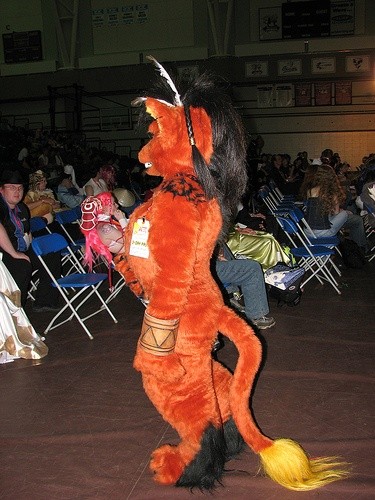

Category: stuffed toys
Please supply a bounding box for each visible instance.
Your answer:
[121,55,352,492]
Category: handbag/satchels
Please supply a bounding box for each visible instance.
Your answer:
[263,261,305,290]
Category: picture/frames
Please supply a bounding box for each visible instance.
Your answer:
[311,57,337,74]
[245,60,269,79]
[277,59,303,76]
[344,55,371,74]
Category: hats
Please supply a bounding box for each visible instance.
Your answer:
[59,172,73,182]
[0,169,27,185]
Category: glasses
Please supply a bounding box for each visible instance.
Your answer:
[4,185,24,192]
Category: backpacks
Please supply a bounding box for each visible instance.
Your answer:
[265,275,303,307]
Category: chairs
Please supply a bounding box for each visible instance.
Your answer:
[19,204,146,339]
[259,180,342,296]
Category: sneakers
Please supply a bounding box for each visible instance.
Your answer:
[248,316,276,330]
[230,297,245,313]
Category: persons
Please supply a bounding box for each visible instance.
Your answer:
[0,117,375,365]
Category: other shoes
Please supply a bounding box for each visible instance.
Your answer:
[31,301,62,313]
